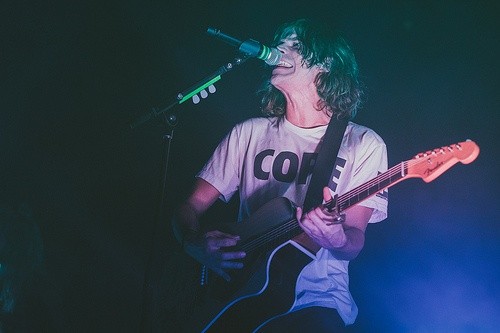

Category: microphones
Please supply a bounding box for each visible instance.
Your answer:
[206,26,281,65]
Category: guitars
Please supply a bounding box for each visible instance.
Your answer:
[181,137,481,331]
